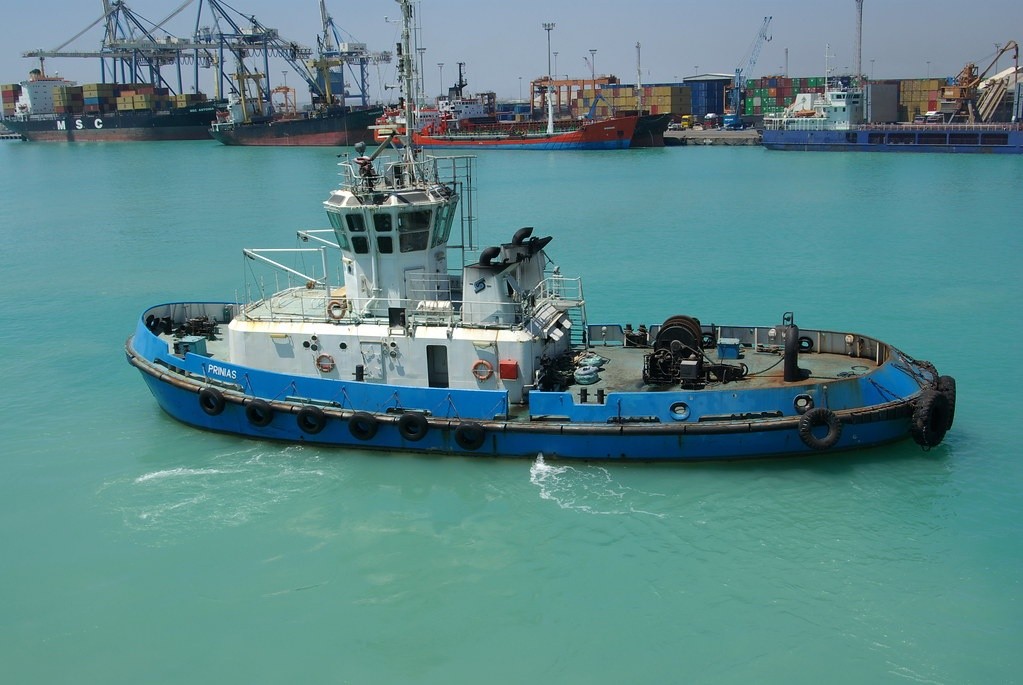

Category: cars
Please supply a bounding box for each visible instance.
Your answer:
[692,122,704,131]
[668,123,684,131]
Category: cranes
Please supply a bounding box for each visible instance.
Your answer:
[20,0,392,116]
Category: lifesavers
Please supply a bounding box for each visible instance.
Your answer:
[347,411,378,441]
[450,137,454,141]
[496,137,500,141]
[797,406,843,451]
[520,136,525,140]
[199,388,225,417]
[546,134,550,139]
[398,412,429,442]
[315,353,335,374]
[245,399,273,428]
[454,420,486,452]
[471,360,493,381]
[912,389,950,450]
[581,127,585,132]
[937,374,957,430]
[296,405,327,434]
[471,137,474,141]
[326,301,345,320]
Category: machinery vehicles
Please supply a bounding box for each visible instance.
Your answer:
[722,14,773,131]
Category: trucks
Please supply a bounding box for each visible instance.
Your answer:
[704,113,723,130]
[680,114,704,130]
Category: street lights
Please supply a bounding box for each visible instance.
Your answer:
[634,40,641,115]
[553,51,558,78]
[437,63,444,95]
[590,49,597,99]
[519,77,522,102]
[416,46,427,96]
[543,23,556,79]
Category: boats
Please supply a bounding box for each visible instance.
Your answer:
[366,0,677,151]
[207,1,400,147]
[124,0,970,462]
[1,0,232,142]
[793,109,816,117]
[757,0,1022,153]
